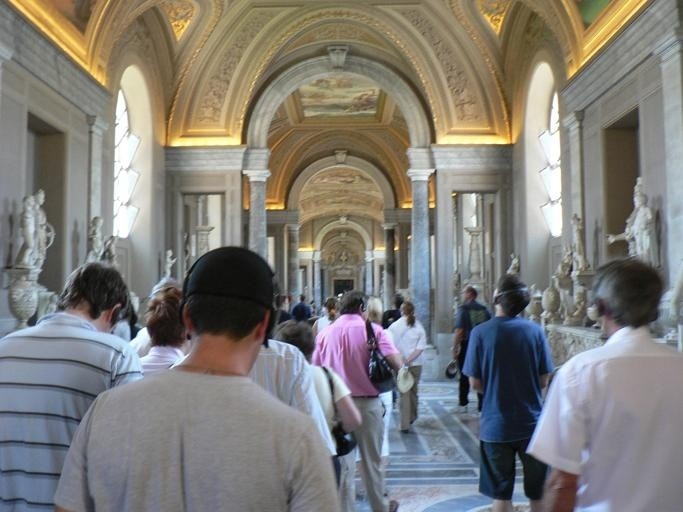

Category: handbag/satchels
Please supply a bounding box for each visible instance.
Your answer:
[332,421,357,455]
[366,317,396,394]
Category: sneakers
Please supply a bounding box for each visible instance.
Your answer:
[389,500,399,512]
[450,406,467,413]
[472,412,482,417]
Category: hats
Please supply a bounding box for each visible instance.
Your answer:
[186,248,279,312]
[149,280,176,298]
[446,360,459,379]
[397,367,414,393]
[367,299,382,323]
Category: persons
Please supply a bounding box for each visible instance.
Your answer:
[5,187,178,279]
[0,245,429,512]
[521,255,683,511]
[570,212,590,272]
[602,174,661,270]
[507,252,520,273]
[461,272,555,512]
[451,284,491,416]
